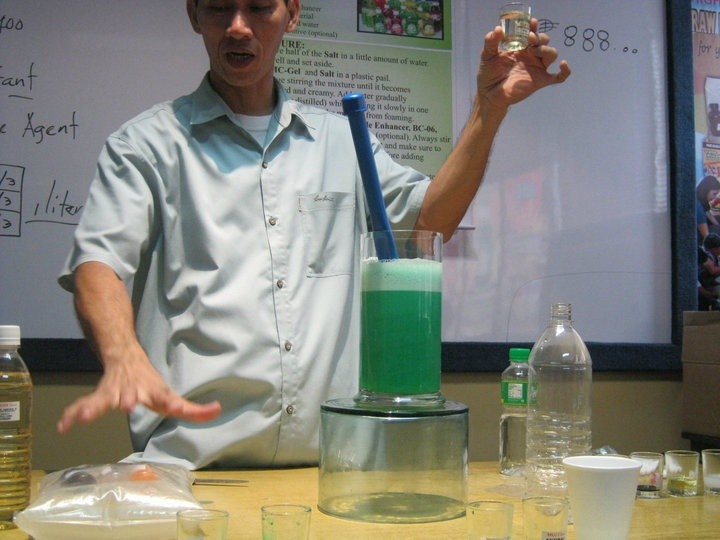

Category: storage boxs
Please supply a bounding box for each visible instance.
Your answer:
[681,311,720,363]
[680,364,720,437]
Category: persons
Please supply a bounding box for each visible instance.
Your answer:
[695,176,720,311]
[56,0,570,470]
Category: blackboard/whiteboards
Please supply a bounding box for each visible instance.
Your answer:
[0,0,698,373]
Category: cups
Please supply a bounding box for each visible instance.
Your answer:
[353,228,448,411]
[562,455,643,540]
[261,504,312,540]
[630,451,664,499]
[522,497,570,540]
[664,449,700,497]
[496,1,532,52]
[316,397,471,523]
[175,508,230,540]
[465,499,514,540]
[701,449,720,496]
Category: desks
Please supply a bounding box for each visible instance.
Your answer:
[0,454,720,540]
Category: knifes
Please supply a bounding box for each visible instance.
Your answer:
[193,478,249,484]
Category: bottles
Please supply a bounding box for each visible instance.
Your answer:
[0,325,34,532]
[499,347,530,478]
[522,303,593,517]
